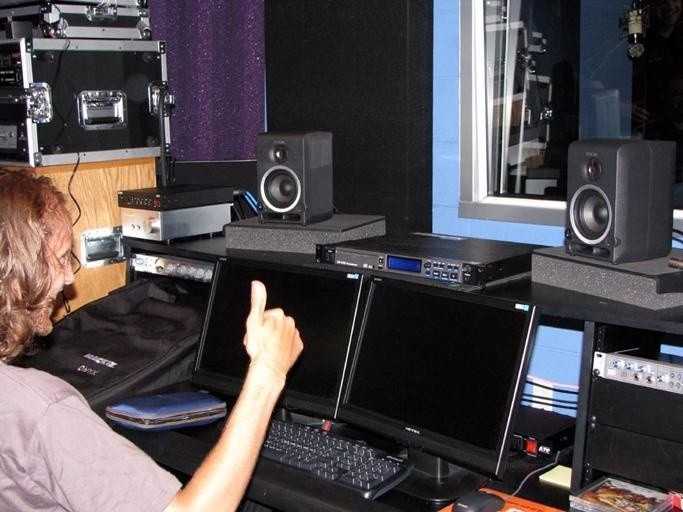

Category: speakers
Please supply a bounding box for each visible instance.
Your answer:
[255,131,334,225]
[564,139,677,265]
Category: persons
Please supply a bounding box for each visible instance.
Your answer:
[0,164,305,512]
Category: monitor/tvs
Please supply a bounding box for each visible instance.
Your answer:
[337,268,543,503]
[189,255,368,422]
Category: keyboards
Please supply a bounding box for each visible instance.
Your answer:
[217,417,416,502]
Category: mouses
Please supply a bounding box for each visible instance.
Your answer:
[452,491,506,512]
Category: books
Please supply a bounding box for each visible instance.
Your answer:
[568,476,674,512]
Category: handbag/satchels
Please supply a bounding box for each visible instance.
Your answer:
[8,272,210,422]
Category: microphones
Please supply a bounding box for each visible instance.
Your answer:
[627,9,648,59]
